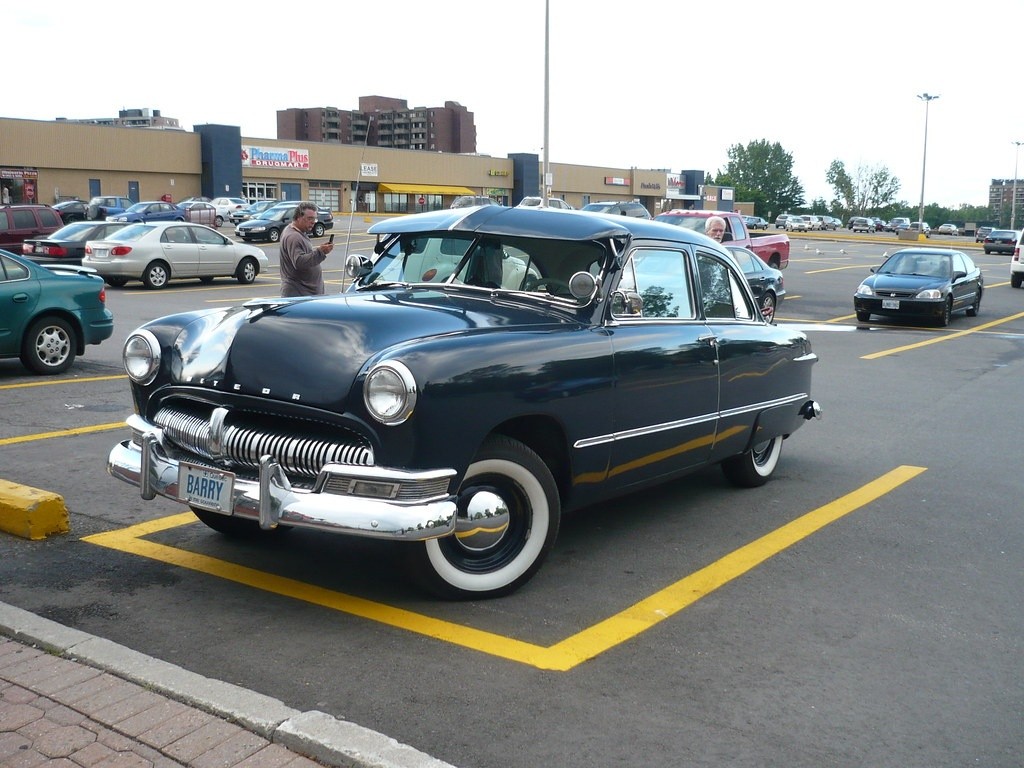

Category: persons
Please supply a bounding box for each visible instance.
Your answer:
[705,216,726,243]
[1,184,10,205]
[279,202,335,298]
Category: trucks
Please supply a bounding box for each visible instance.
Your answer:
[884,218,910,233]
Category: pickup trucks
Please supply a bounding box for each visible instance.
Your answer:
[650,209,790,270]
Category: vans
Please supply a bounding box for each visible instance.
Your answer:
[907,222,931,238]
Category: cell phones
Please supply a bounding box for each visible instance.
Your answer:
[328,234,334,244]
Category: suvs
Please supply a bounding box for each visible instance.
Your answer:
[450,195,501,209]
[0,203,65,257]
[84,196,135,221]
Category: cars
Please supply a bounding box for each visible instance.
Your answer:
[249,201,333,220]
[514,196,575,210]
[976,227,997,243]
[107,202,823,598]
[869,217,880,222]
[51,200,89,226]
[210,197,251,214]
[741,214,843,233]
[82,220,269,290]
[106,200,186,223]
[854,247,984,327]
[234,205,334,242]
[0,249,114,374]
[22,217,134,270]
[896,224,910,235]
[983,230,1022,255]
[939,224,957,236]
[853,218,876,233]
[177,202,229,228]
[578,201,653,220]
[1010,227,1024,288]
[874,220,886,232]
[723,245,786,324]
[847,217,862,230]
[229,201,281,227]
[176,196,212,204]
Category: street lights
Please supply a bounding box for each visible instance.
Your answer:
[1006,139,1024,230]
[917,93,939,233]
